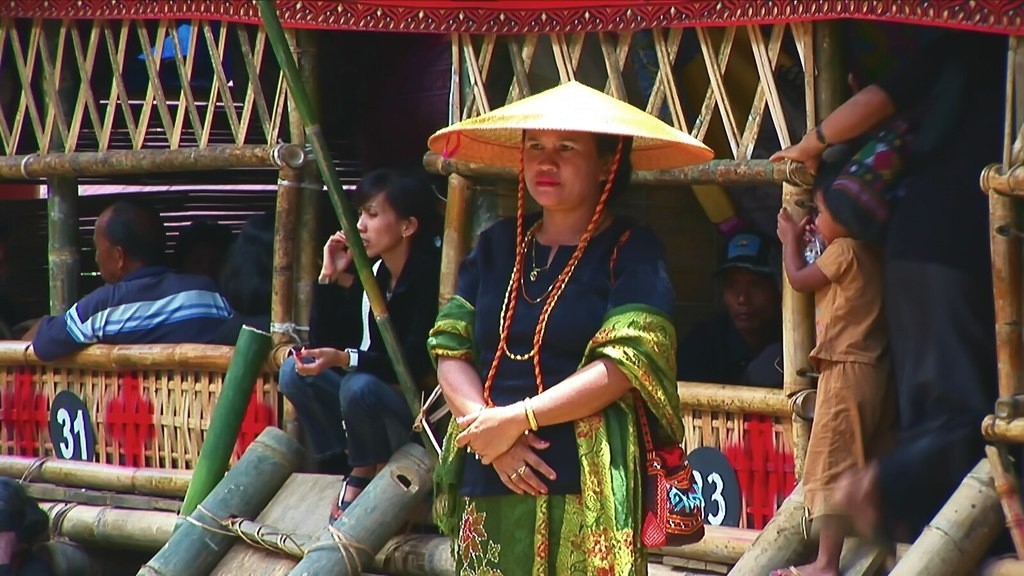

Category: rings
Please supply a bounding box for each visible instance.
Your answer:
[475,451,482,460]
[466,445,475,454]
[518,464,527,475]
[510,471,519,479]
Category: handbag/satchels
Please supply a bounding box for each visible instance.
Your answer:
[633,385,706,547]
[820,107,920,242]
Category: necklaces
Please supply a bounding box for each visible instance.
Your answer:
[498,209,610,361]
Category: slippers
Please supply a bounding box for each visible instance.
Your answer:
[328,466,376,528]
[768,566,840,576]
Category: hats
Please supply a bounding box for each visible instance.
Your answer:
[427,78,716,172]
[714,228,783,284]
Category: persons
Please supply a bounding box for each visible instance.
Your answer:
[20,196,241,362]
[176,220,273,317]
[768,161,899,576]
[276,165,442,526]
[425,91,686,576]
[673,231,783,389]
[769,17,1024,555]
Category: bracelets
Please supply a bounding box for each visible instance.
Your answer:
[524,397,539,431]
[815,120,835,147]
[342,347,359,371]
